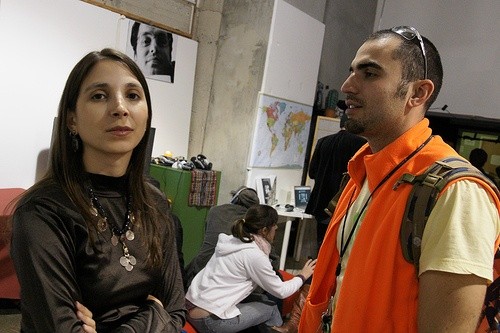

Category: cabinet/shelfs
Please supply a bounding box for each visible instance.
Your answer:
[150,164,222,270]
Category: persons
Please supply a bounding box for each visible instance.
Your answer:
[304,111,368,254]
[469,147,498,186]
[130,22,176,83]
[4,48,189,333]
[297,26,500,333]
[183,186,317,333]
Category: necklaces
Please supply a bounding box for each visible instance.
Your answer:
[88,186,136,272]
[317,133,437,333]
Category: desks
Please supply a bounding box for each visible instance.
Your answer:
[275,205,314,271]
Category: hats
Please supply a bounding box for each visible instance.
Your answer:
[230,186,259,207]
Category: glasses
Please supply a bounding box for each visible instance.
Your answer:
[391,25,427,80]
